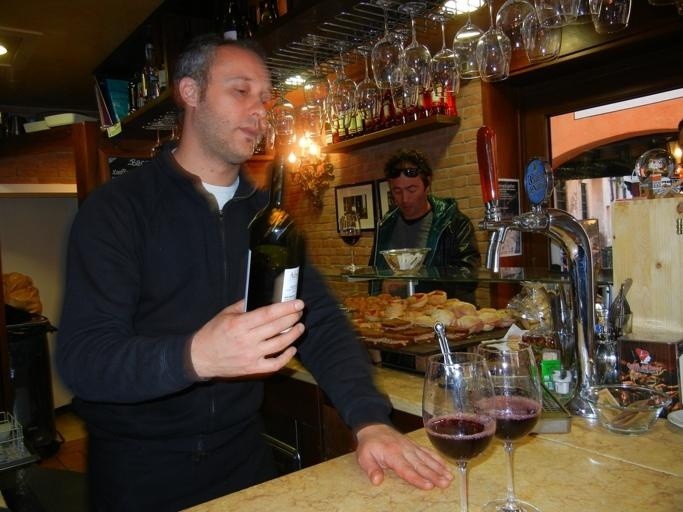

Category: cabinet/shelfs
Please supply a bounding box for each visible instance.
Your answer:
[323,269,612,380]
[321,401,425,464]
[88,0,440,132]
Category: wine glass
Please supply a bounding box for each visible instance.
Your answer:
[152,69,326,159]
[339,215,362,270]
[475,341,540,511]
[303,1,460,118]
[453,0,629,83]
[421,351,498,511]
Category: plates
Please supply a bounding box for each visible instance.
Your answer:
[667,409,683,427]
[23,113,98,134]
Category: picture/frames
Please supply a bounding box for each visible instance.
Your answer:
[287,132,334,206]
[335,179,376,233]
[378,179,397,218]
[496,179,522,256]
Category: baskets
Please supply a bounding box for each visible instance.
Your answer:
[0,411,24,464]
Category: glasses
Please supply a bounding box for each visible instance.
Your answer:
[383,168,418,178]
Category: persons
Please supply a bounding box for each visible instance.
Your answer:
[49,33,454,511]
[367,148,481,383]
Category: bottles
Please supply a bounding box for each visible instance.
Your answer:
[11,115,23,136]
[243,133,302,359]
[332,78,457,144]
[128,1,293,114]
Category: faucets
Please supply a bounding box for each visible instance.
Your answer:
[478,209,602,418]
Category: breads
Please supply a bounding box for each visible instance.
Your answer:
[335,290,517,350]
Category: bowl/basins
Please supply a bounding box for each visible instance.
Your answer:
[580,384,672,433]
[379,247,432,274]
